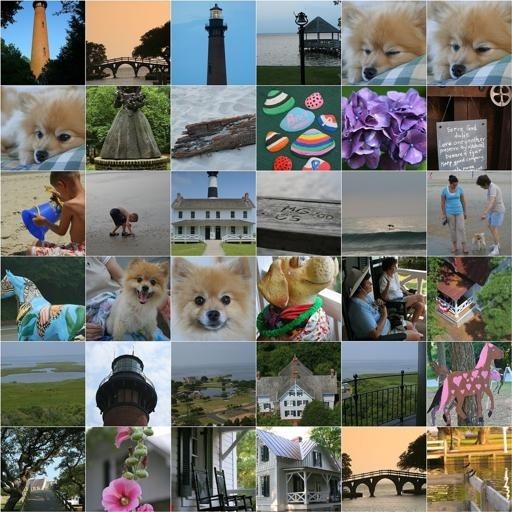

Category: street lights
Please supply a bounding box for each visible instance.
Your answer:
[295,11,308,85]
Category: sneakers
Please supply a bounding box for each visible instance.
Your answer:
[487,246,500,256]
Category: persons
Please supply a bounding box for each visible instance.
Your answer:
[85,257,170,341]
[109,207,138,236]
[441,175,470,254]
[344,264,420,341]
[101,86,161,159]
[378,257,425,328]
[476,175,505,256]
[25,171,85,257]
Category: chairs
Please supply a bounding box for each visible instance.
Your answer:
[343,289,406,341]
[191,463,253,511]
[370,256,416,319]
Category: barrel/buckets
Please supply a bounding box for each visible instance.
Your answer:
[22,203,59,240]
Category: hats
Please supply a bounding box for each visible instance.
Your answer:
[344,264,370,299]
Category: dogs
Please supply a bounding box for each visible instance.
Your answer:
[341,1,426,85]
[1,85,86,167]
[171,257,256,340]
[103,257,170,340]
[428,0,511,85]
[470,232,486,250]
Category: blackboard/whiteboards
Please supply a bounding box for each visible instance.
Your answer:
[436,119,488,170]
